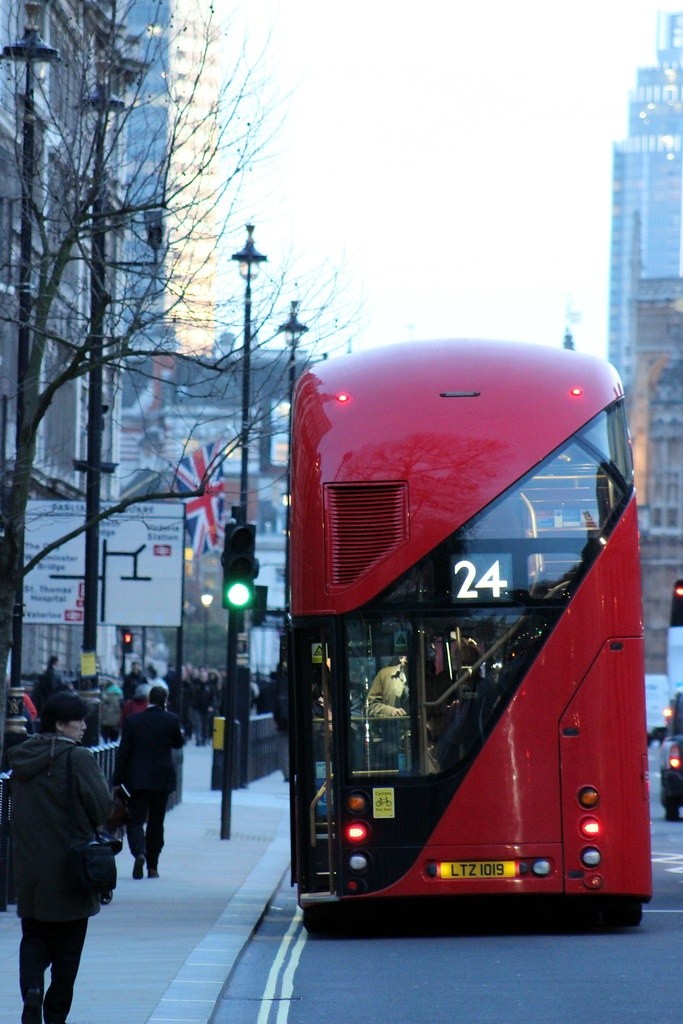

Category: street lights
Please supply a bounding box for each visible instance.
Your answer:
[278,297,308,629]
[1,3,62,762]
[81,60,124,758]
[215,221,268,842]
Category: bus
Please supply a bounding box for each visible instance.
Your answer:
[255,337,657,931]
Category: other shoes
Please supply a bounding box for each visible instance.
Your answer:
[132,854,145,879]
[148,868,159,878]
[21,988,43,1024]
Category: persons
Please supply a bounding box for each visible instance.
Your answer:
[6,692,114,1024]
[0,654,288,782]
[113,686,186,881]
[312,632,487,768]
[365,654,411,771]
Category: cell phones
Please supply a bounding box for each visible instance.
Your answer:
[114,783,131,801]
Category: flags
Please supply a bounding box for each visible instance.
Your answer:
[168,438,230,562]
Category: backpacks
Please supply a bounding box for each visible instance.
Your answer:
[101,699,122,726]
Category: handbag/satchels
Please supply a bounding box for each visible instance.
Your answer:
[96,822,125,855]
[80,843,118,889]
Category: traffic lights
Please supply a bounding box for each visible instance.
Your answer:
[221,522,258,612]
[120,628,134,653]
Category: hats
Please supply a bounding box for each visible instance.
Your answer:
[133,684,151,698]
[105,684,123,696]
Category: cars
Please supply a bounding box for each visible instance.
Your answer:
[644,577,682,822]
